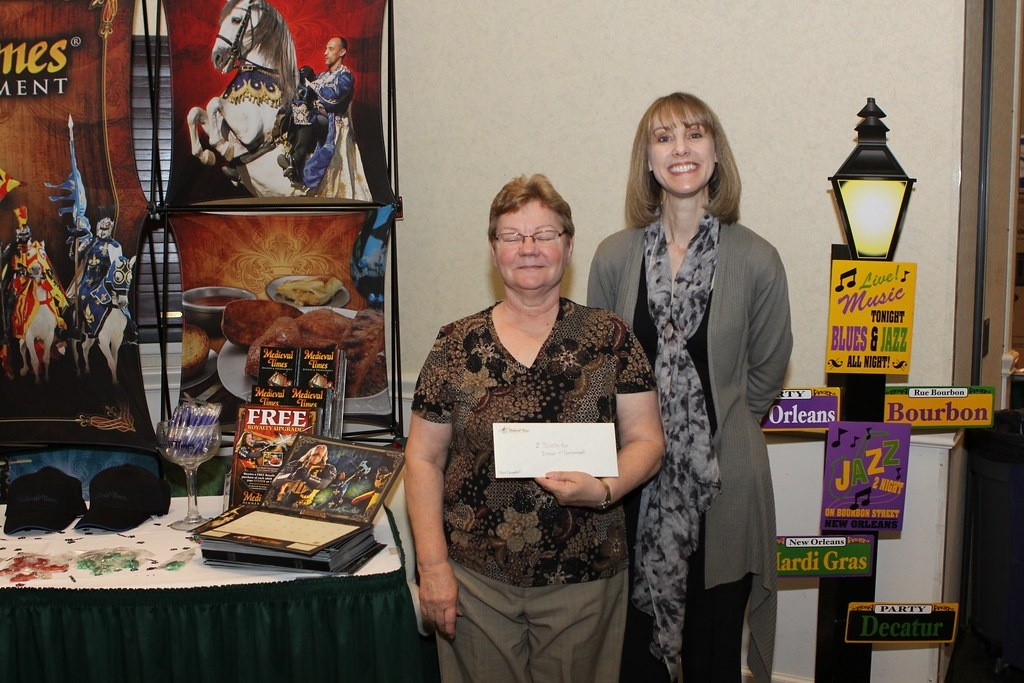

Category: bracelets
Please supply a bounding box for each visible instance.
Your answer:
[599,478,611,509]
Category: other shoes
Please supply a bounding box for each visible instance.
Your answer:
[278,153,303,180]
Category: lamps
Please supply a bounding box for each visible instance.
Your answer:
[828,96,915,262]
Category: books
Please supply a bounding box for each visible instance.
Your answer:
[187,335,403,576]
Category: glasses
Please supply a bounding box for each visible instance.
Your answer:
[495,229,567,246]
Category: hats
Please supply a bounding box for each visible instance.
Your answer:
[75,462,172,531]
[4,465,88,534]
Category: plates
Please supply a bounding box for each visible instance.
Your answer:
[217,339,393,417]
[341,381,388,402]
[179,349,221,392]
[265,274,349,312]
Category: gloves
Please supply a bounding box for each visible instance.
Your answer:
[299,66,317,83]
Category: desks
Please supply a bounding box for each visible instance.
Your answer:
[0,494,440,683]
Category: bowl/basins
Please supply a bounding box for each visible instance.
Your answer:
[182,284,256,327]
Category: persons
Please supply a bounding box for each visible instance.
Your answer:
[403,170,665,683]
[586,92,796,683]
[278,35,356,191]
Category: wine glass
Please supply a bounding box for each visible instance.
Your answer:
[156,420,221,532]
